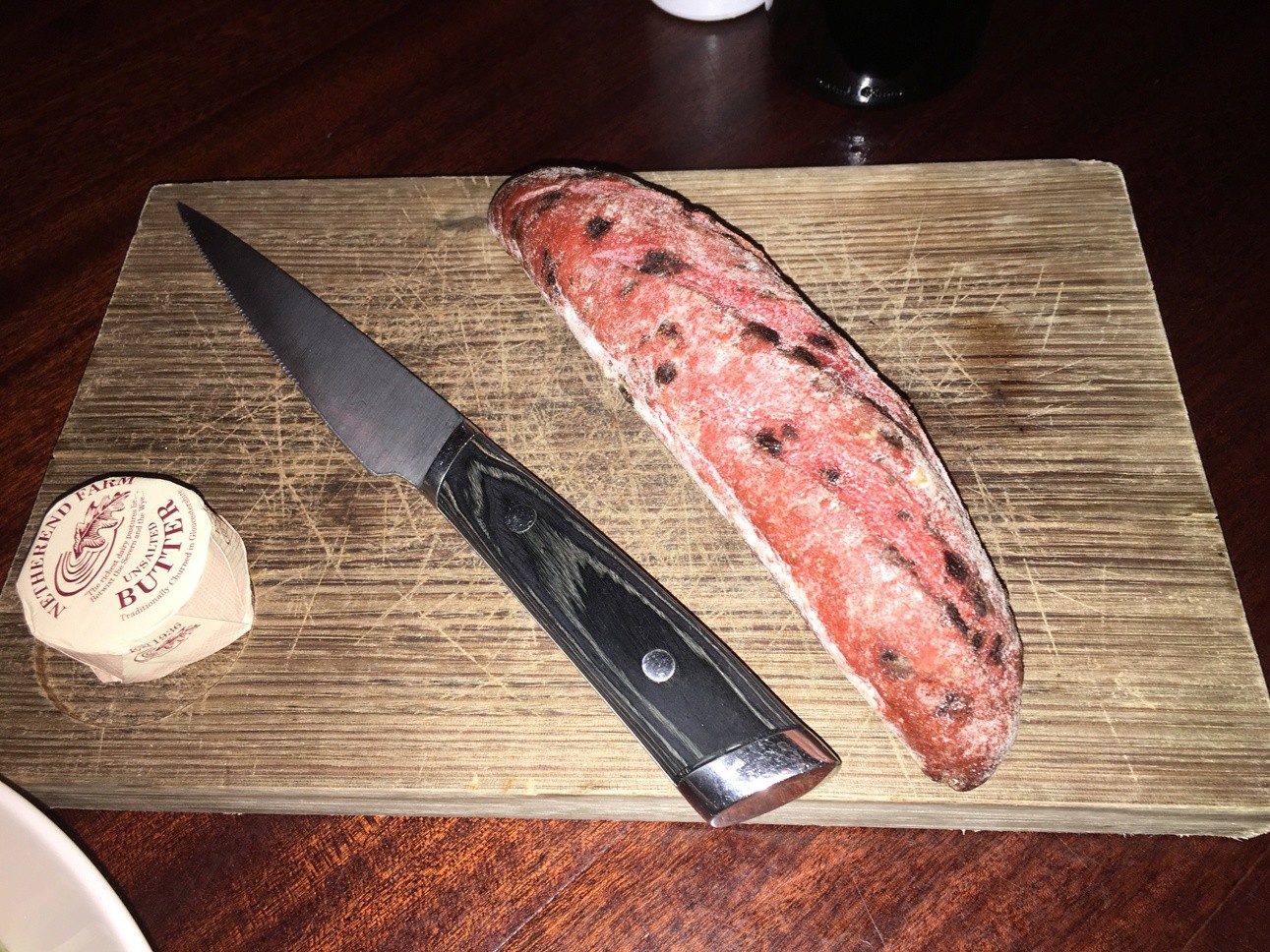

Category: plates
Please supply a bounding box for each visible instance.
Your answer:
[1,783,153,952]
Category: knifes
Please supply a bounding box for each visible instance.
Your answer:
[177,200,843,829]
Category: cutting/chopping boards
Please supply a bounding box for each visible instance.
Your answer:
[1,161,1270,846]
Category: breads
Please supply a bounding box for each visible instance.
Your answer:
[488,166,1022,792]
